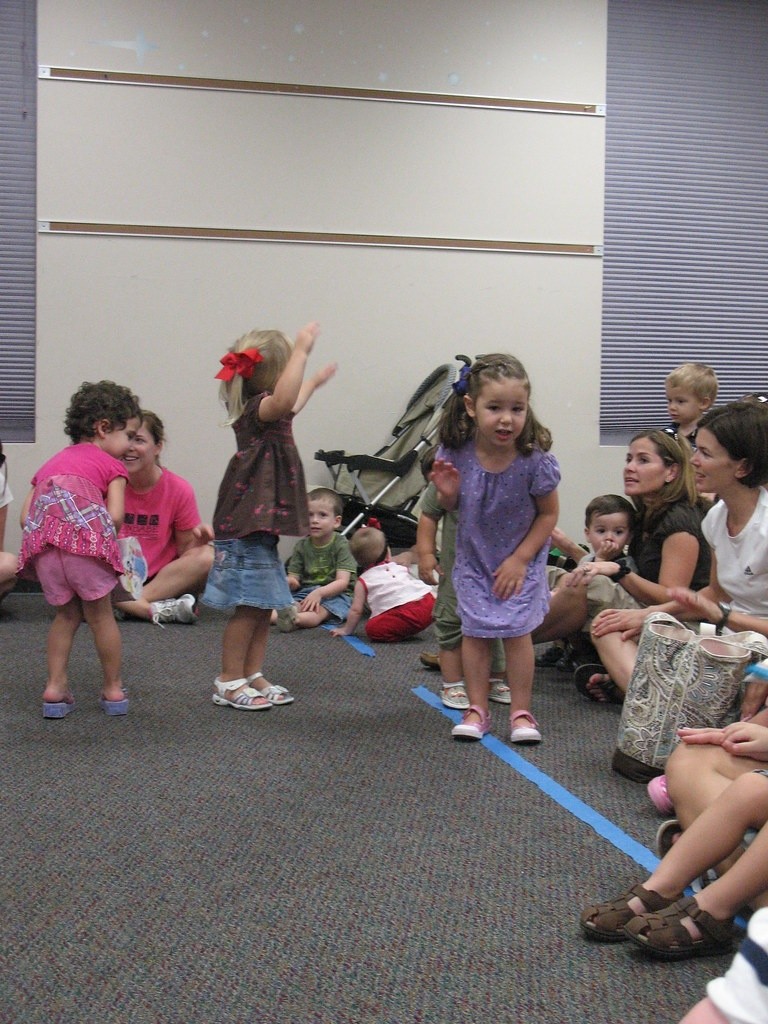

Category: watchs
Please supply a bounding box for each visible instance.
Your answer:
[715,601,731,631]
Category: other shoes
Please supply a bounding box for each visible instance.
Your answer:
[452,704,492,739]
[489,680,512,703]
[440,686,470,709]
[420,652,441,669]
[276,606,299,632]
[509,709,541,742]
[536,641,599,673]
[648,775,675,816]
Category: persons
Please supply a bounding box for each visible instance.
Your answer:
[665,363,718,500]
[198,322,334,710]
[390,443,443,591]
[330,526,437,643]
[534,494,639,673]
[590,392,768,690]
[429,352,561,742]
[420,429,710,667]
[15,379,143,718]
[270,488,357,632]
[416,481,511,709]
[580,656,768,1024]
[102,412,214,629]
[0,442,19,603]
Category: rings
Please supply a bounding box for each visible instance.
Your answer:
[581,566,588,572]
[696,595,700,600]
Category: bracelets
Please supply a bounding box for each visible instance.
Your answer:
[609,566,631,582]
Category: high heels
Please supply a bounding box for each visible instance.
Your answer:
[97,689,129,715]
[43,691,75,718]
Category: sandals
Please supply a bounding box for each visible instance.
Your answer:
[574,663,625,705]
[246,672,294,705]
[656,819,758,890]
[579,884,684,941]
[623,895,734,959]
[212,677,273,710]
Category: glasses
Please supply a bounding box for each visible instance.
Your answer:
[660,427,678,442]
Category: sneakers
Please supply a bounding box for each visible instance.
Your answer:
[113,606,129,622]
[152,594,199,628]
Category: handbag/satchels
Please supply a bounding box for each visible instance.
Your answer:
[613,612,768,783]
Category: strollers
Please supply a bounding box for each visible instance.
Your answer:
[285,355,471,575]
